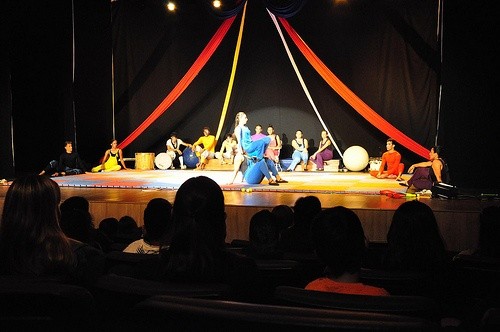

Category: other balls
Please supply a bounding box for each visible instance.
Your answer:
[343,146,369,172]
[183,147,199,168]
[154,152,172,169]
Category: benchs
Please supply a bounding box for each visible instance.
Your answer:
[196,159,340,172]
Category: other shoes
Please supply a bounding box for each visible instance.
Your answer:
[276,180,288,183]
[399,183,409,187]
[269,183,279,185]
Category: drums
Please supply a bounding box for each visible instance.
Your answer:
[369,157,382,172]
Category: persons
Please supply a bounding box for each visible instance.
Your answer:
[229,149,288,186]
[215,132,237,164]
[92,139,128,172]
[233,111,271,160]
[266,124,282,163]
[369,138,404,181]
[380,201,449,299]
[309,130,334,171]
[303,207,391,297]
[254,124,263,134]
[192,127,217,170]
[399,144,447,189]
[99,197,321,260]
[286,130,309,171]
[156,176,257,285]
[166,134,192,170]
[59,196,106,254]
[0,174,106,284]
[241,209,302,287]
[38,140,83,177]
